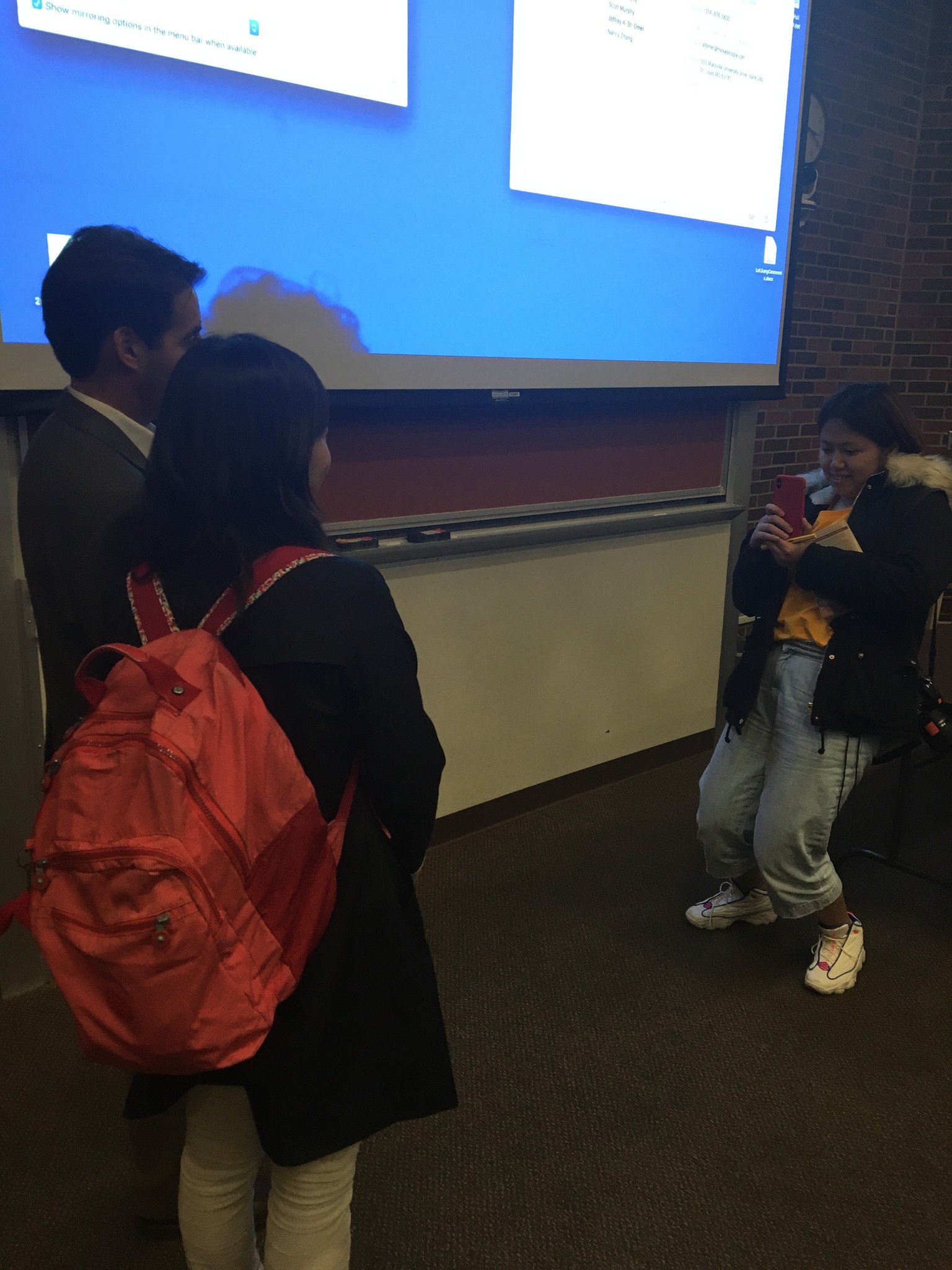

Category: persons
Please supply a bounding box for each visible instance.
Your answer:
[101,334,464,1269]
[19,226,207,762]
[686,385,952,994]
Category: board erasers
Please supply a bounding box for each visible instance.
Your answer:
[336,536,378,552]
[407,528,451,544]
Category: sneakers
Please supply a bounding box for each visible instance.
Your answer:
[685,878,778,930]
[804,911,866,995]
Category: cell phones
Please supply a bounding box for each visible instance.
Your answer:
[775,474,804,539]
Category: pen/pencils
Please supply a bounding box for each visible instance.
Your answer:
[761,533,817,549]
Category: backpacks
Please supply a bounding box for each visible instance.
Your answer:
[0,544,360,1074]
